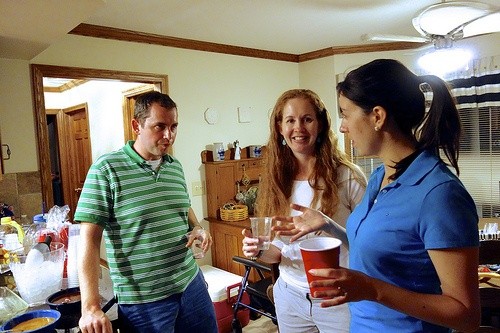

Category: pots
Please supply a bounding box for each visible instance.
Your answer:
[45,286,82,313]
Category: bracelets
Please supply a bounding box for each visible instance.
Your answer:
[192,225,204,229]
[256,250,262,258]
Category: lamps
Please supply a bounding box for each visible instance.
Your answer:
[415,38,472,75]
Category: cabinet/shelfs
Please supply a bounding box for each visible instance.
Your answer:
[202,156,270,283]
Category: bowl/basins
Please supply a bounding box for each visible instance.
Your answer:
[0,310,61,333]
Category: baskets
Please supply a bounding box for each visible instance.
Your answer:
[220,205,248,221]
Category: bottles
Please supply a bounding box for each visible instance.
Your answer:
[0,215,59,264]
[27,234,53,265]
[213,142,225,161]
[249,145,262,157]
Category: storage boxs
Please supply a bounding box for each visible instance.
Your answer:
[199,264,250,333]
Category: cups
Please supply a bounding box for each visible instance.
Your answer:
[248,216,273,251]
[298,237,342,300]
[185,229,211,260]
[65,223,84,288]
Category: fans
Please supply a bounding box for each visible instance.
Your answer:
[371,0,500,51]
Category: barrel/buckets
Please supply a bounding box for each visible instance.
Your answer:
[5,243,65,303]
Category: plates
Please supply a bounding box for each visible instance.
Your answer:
[0,285,28,326]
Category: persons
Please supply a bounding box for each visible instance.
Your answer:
[73,92,218,333]
[271,59,481,333]
[242,89,367,333]
